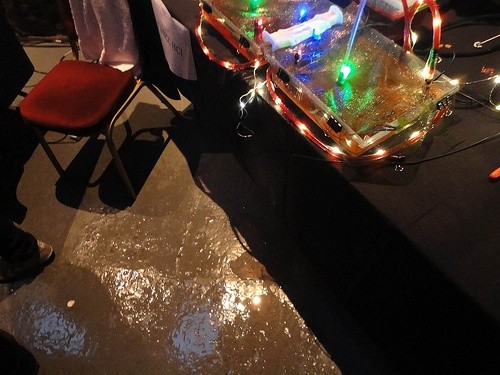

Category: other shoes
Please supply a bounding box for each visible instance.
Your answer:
[1,236,53,284]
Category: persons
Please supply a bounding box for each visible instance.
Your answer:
[0,18,54,279]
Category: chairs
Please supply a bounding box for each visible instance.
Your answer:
[20,0,181,200]
